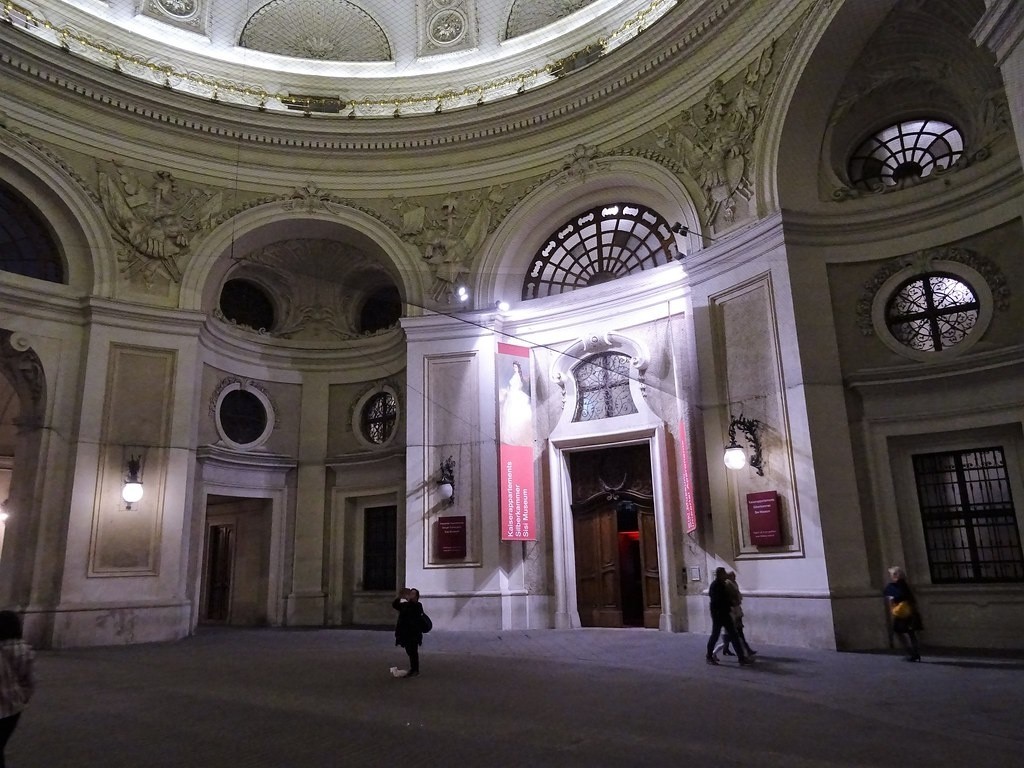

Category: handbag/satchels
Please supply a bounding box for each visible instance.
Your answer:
[417,613,432,633]
[892,602,911,617]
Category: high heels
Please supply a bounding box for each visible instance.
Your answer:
[748,650,757,657]
[723,649,734,656]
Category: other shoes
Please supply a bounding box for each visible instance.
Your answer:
[904,654,919,661]
[740,657,754,666]
[405,672,421,678]
[706,656,718,665]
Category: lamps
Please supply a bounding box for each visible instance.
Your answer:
[494,300,509,311]
[675,244,686,261]
[121,454,144,510]
[724,413,765,478]
[436,456,456,505]
[456,283,469,302]
[669,222,689,237]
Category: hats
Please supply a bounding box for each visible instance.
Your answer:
[888,567,905,578]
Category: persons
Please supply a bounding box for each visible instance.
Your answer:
[0,611,38,768]
[883,566,924,663]
[392,587,425,676]
[706,567,758,665]
